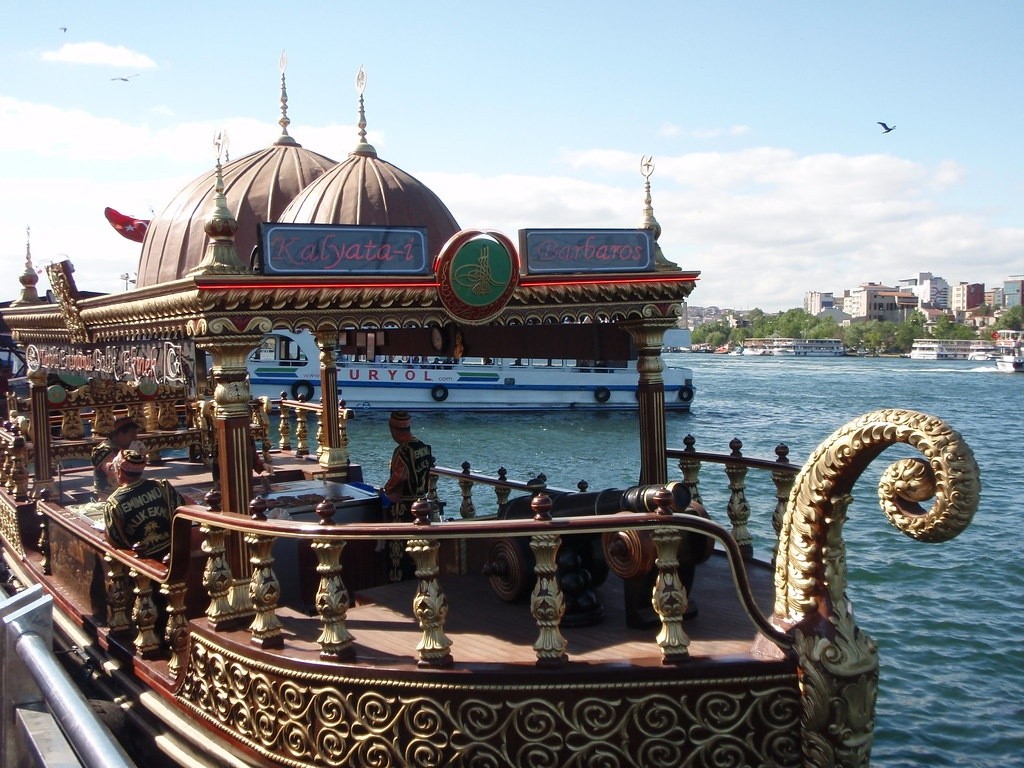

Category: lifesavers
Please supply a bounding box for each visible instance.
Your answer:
[679,388,693,401]
[595,387,610,402]
[291,380,315,402]
[431,384,449,402]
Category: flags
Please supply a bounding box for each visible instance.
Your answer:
[105,207,151,243]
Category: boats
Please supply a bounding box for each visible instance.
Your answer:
[200,326,699,420]
[910,338,1002,361]
[0,55,987,768]
[661,337,881,358]
[993,329,1024,373]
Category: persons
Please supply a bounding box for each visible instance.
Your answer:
[104,449,190,640]
[383,353,453,370]
[91,419,140,503]
[385,411,432,582]
[211,434,273,492]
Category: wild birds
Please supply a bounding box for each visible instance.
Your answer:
[60,27,140,81]
[877,122,896,134]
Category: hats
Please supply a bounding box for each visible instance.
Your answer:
[115,449,145,473]
[110,416,135,432]
[389,411,411,428]
